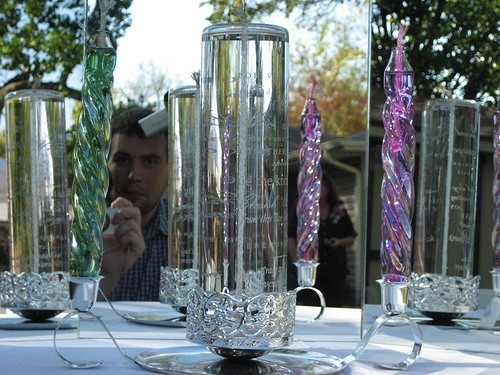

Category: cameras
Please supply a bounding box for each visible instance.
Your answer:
[101,208,127,240]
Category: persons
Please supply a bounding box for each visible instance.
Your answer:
[286,175,357,307]
[97,107,169,302]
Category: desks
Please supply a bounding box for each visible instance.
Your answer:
[0,300,499,374]
